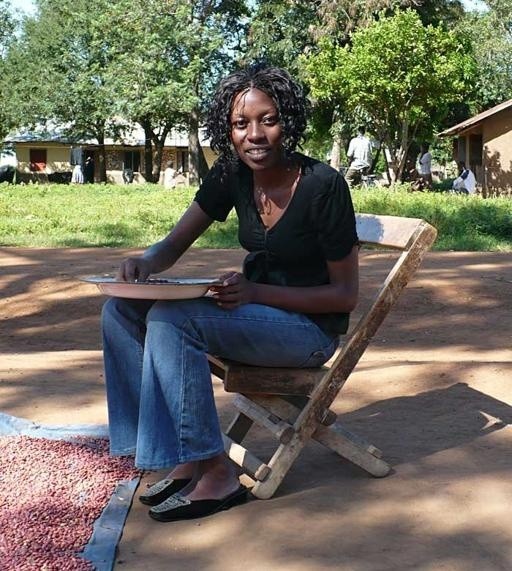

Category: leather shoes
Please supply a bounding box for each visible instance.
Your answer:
[140,477,192,506]
[149,480,248,522]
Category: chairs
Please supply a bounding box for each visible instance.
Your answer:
[207,210,437,503]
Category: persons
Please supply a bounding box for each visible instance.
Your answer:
[453,162,477,194]
[81,156,95,184]
[344,123,380,190]
[415,143,434,186]
[162,159,183,191]
[102,62,362,522]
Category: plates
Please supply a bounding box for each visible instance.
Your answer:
[79,275,223,299]
